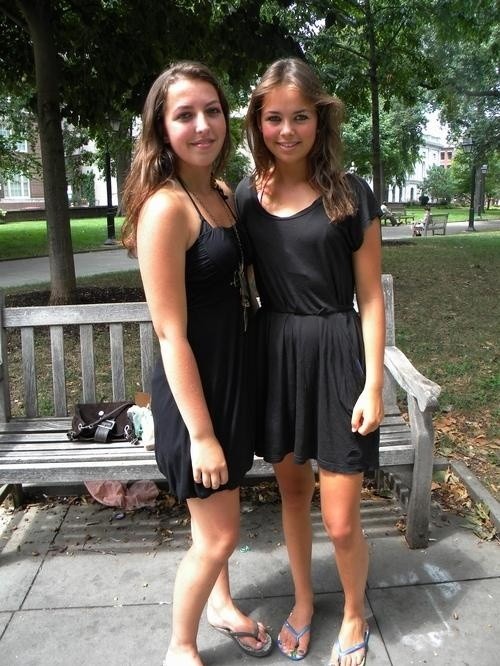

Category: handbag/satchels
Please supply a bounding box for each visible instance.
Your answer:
[68,400,139,445]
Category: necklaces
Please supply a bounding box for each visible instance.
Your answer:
[176,170,251,333]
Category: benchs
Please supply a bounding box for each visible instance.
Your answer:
[379,208,414,225]
[411,213,449,236]
[0,275,443,548]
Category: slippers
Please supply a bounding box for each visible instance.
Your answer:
[331,624,369,666]
[210,620,273,657]
[278,620,311,661]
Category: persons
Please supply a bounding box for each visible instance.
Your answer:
[115,61,277,666]
[379,201,403,226]
[412,207,432,237]
[236,60,386,666]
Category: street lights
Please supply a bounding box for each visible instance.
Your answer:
[100,110,122,246]
[462,136,489,231]
